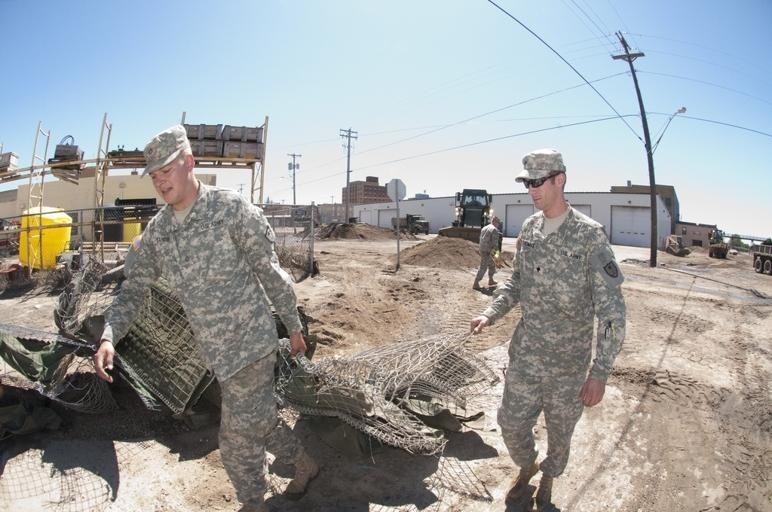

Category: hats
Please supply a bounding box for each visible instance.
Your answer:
[140,125,189,180]
[515,150,566,183]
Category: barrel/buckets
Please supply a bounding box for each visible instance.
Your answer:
[124,206,140,242]
[18,206,73,270]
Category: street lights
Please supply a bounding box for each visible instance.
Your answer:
[643,107,691,268]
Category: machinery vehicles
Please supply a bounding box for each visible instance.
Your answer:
[438,187,504,254]
[707,228,729,259]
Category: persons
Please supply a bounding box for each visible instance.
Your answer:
[473,217,501,289]
[96,126,319,512]
[471,147,625,508]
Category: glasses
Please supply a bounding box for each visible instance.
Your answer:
[523,172,563,188]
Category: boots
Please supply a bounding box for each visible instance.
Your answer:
[535,475,553,506]
[504,463,539,499]
[286,452,319,500]
[237,497,265,512]
[489,278,498,285]
[473,281,483,289]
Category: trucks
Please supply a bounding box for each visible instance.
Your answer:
[389,213,430,236]
[752,242,772,275]
